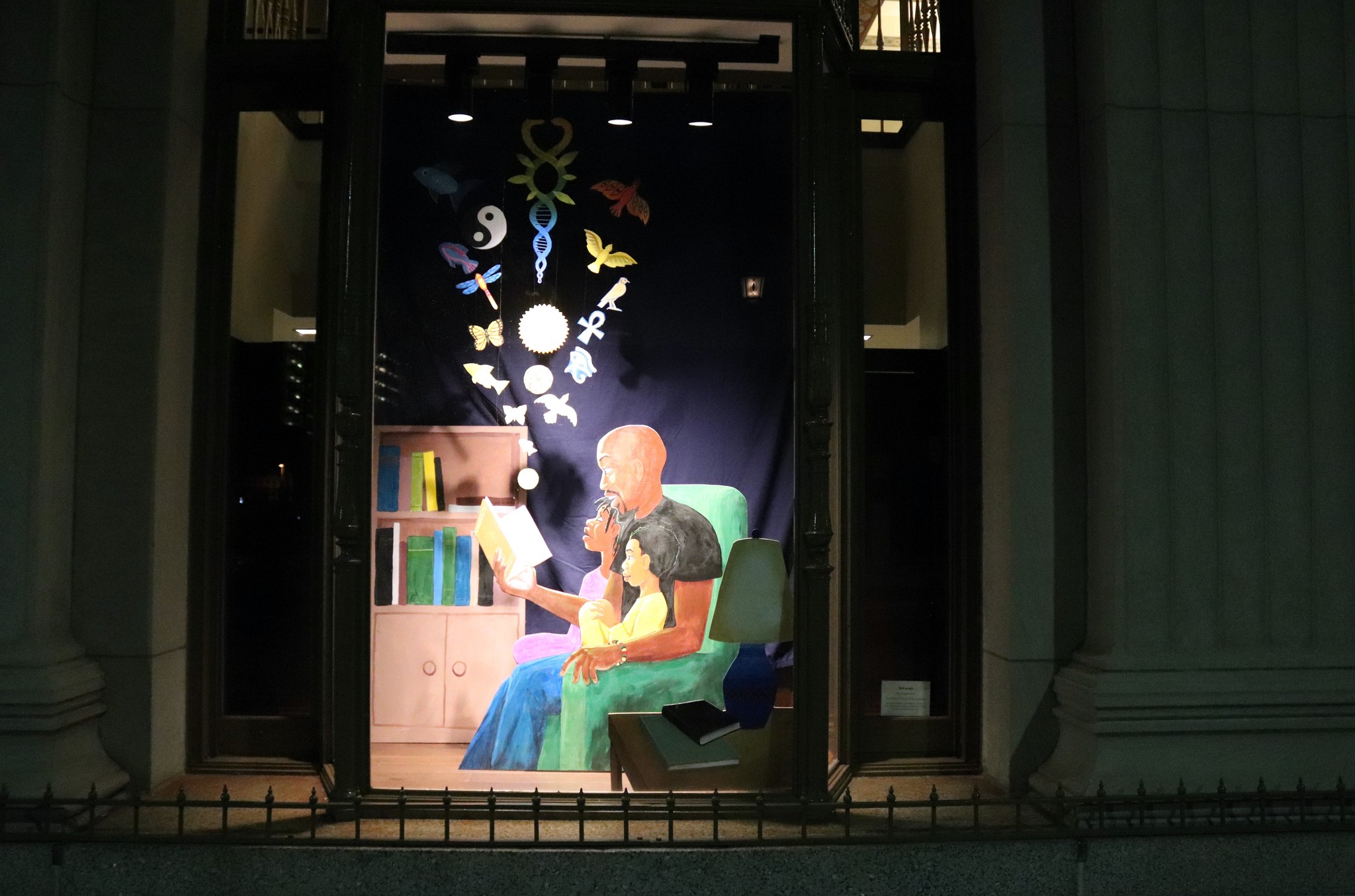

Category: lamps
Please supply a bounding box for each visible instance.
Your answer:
[445,54,480,122]
[525,56,558,121]
[604,58,638,125]
[684,60,718,127]
